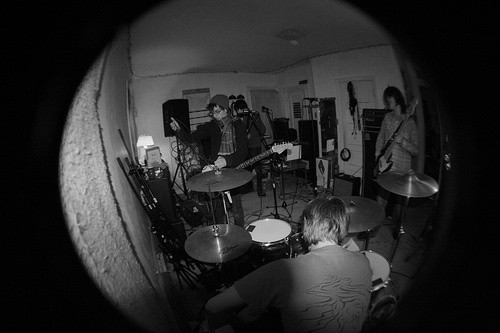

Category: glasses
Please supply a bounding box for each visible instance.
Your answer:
[210,108,224,115]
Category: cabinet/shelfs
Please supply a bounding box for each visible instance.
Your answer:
[149,168,178,224]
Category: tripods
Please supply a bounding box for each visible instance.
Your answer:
[266,153,297,217]
[172,137,192,201]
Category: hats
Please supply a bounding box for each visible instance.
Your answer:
[209,94,229,110]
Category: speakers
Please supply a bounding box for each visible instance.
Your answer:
[272,119,289,141]
[162,99,190,136]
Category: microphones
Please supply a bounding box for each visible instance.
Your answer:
[262,106,272,112]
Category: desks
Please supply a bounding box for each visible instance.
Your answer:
[270,161,307,200]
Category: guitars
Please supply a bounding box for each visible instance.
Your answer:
[373,96,419,182]
[202,141,295,197]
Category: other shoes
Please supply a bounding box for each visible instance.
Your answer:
[258,192,266,198]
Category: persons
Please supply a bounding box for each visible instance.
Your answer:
[204,196,373,333]
[170,94,247,229]
[234,100,266,197]
[375,86,419,236]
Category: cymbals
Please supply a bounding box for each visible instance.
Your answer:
[376,169,441,198]
[185,222,250,263]
[340,194,384,232]
[186,165,254,193]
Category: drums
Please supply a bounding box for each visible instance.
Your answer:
[245,218,292,261]
[337,234,368,253]
[359,250,391,300]
